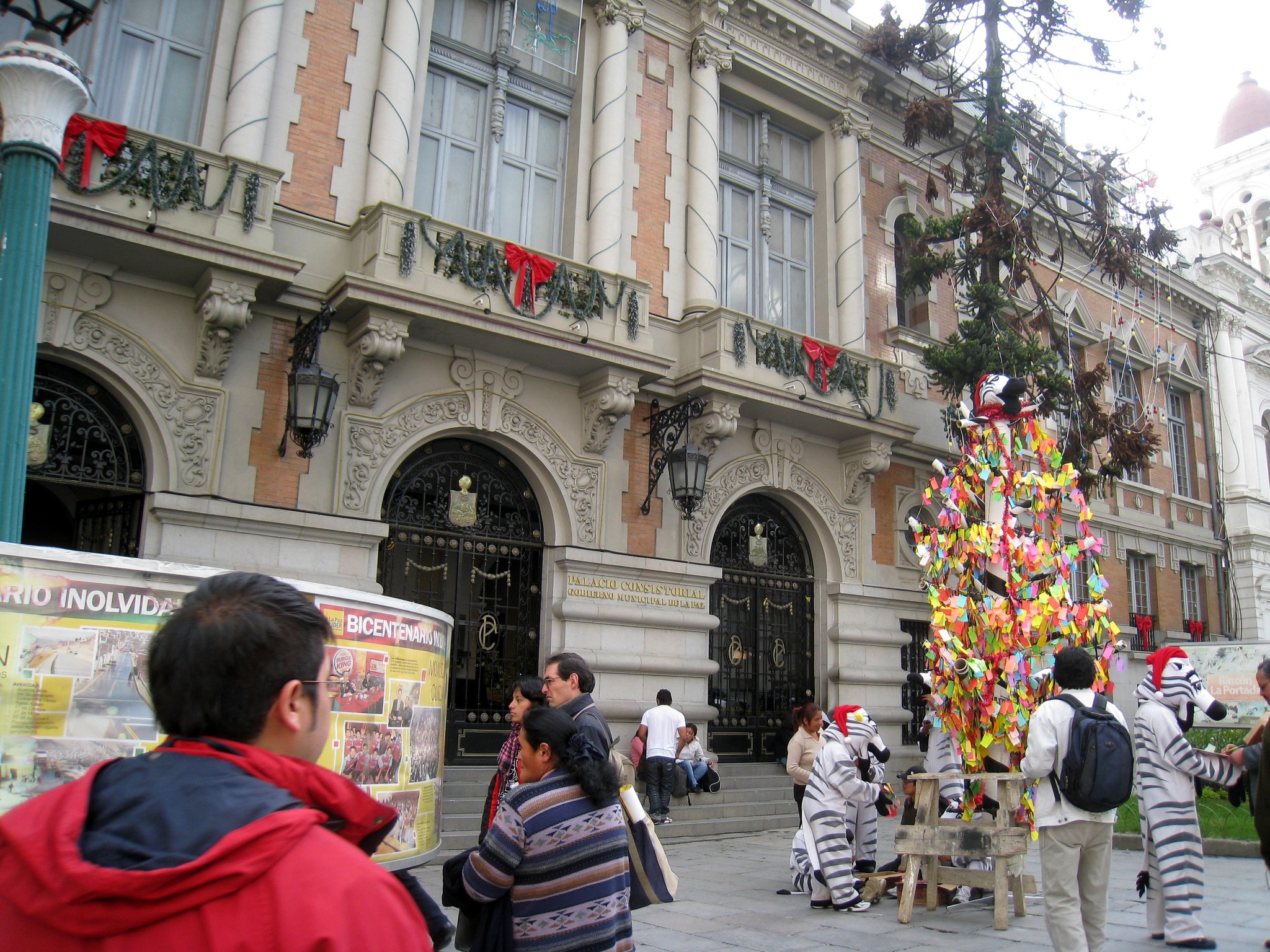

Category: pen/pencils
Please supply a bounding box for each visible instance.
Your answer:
[1223,744,1244,753]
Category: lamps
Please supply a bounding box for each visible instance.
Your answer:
[279,302,347,459]
[641,392,710,521]
[146,204,158,234]
[473,293,491,314]
[848,399,872,420]
[784,380,807,400]
[569,319,589,344]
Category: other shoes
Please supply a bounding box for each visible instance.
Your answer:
[859,877,886,903]
[694,784,703,793]
[859,866,875,873]
[651,816,673,825]
[1166,937,1217,950]
[1151,934,1164,940]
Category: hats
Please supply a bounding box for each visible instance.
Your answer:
[833,704,869,743]
[1145,646,1189,701]
[897,766,927,782]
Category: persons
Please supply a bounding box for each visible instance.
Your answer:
[391,652,715,952]
[1223,657,1270,947]
[785,703,826,829]
[1019,647,1129,952]
[878,671,994,905]
[0,573,434,952]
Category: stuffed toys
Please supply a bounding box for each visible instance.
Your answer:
[1135,647,1246,950]
[791,704,891,912]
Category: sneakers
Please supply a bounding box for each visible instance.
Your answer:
[839,900,872,911]
[952,885,970,903]
[886,886,897,896]
[811,906,833,908]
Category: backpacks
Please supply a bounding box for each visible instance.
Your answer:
[673,761,687,798]
[1041,691,1135,812]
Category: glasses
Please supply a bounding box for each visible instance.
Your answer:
[543,677,561,687]
[262,675,345,720]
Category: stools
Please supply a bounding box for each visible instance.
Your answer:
[894,772,1028,932]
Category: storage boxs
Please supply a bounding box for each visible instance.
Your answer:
[855,871,959,905]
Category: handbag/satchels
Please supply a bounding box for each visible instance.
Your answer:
[617,784,679,912]
[697,759,721,793]
